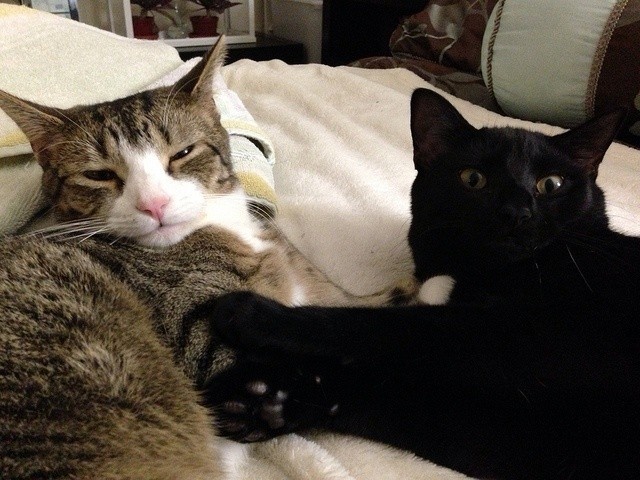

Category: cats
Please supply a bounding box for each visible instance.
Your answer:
[0,34,456,480]
[200,88,640,480]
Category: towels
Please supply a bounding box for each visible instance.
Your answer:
[218,59,639,299]
[0,1,210,160]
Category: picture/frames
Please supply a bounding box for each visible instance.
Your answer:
[123,0,257,48]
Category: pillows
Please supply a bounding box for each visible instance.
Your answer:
[390,0,639,136]
